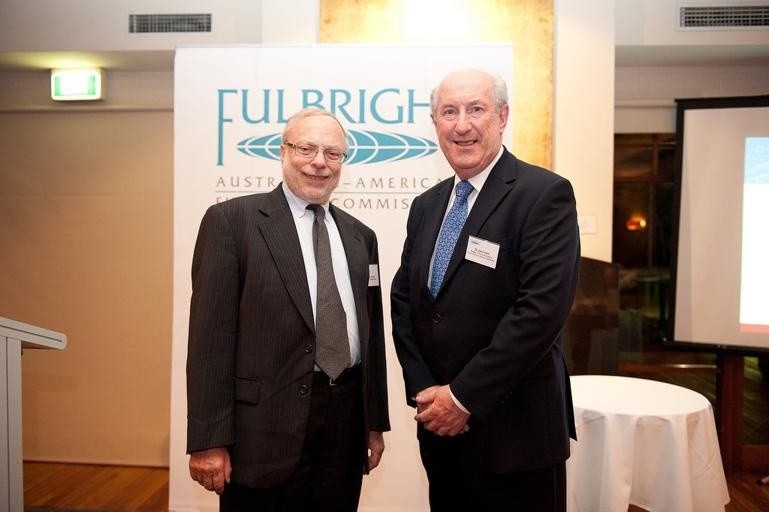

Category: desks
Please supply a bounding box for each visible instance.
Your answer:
[570,374,710,512]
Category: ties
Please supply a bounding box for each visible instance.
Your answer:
[428,181,472,300]
[305,205,352,382]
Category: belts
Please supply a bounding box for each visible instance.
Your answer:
[313,369,352,387]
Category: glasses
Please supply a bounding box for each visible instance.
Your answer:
[287,143,348,165]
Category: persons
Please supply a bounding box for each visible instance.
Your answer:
[386,66,586,511]
[185,106,392,512]
